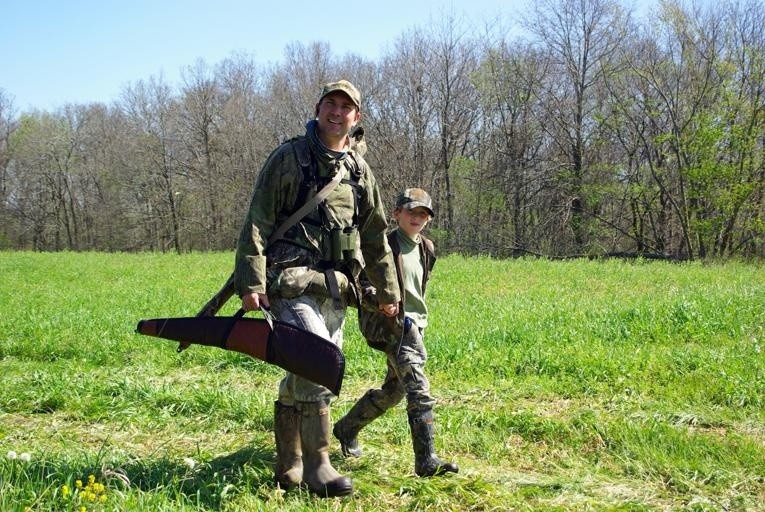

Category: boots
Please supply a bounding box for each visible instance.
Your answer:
[407,408,458,476]
[333,388,386,457]
[274,400,353,498]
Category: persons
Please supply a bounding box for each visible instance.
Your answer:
[232,79,402,497]
[330,187,459,478]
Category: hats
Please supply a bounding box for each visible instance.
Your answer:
[395,187,435,217]
[319,79,361,112]
